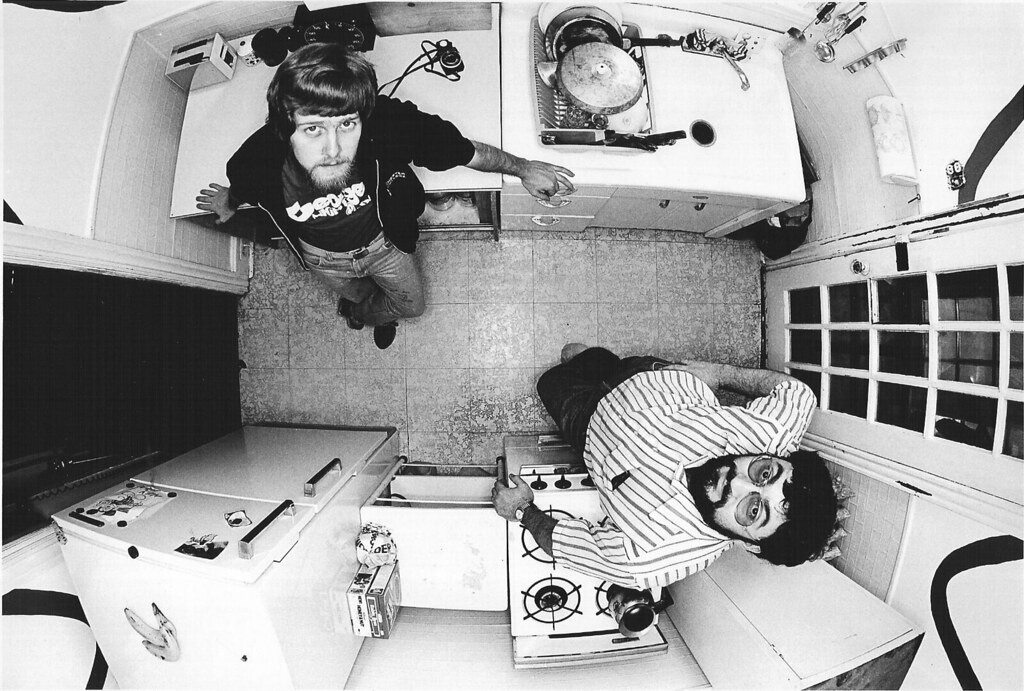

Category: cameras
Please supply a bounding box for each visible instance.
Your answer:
[436,39,464,75]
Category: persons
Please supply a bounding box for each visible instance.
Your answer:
[491,341,836,591]
[194,43,575,348]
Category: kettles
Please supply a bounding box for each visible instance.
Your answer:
[604,585,674,639]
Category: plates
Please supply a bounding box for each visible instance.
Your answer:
[841,36,907,76]
[537,0,623,35]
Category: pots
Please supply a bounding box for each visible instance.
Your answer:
[544,5,685,63]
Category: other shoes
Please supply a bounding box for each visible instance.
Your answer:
[336,298,364,329]
[374,321,398,349]
[561,343,589,364]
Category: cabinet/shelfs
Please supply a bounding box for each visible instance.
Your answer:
[664,539,927,691]
[588,186,781,235]
[500,182,617,234]
[359,455,510,612]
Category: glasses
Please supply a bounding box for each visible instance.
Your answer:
[734,451,773,541]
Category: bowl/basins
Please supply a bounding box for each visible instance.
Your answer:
[426,193,477,211]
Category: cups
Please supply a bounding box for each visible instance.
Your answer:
[236,41,260,67]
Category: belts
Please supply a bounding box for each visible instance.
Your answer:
[297,236,385,260]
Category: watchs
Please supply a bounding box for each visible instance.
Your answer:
[514,501,534,522]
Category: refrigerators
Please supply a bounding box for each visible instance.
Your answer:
[49,424,400,691]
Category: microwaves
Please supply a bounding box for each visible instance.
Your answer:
[162,31,238,93]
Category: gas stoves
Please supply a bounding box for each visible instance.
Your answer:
[509,461,672,671]
